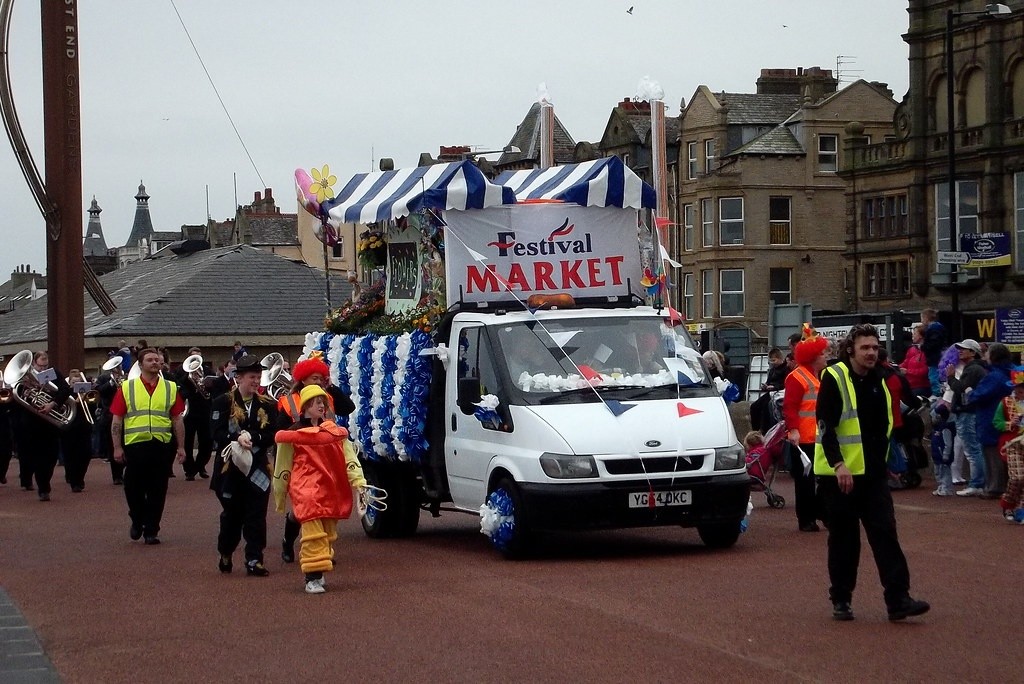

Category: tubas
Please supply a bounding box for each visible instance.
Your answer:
[102,356,128,387]
[259,353,294,402]
[3,350,77,427]
[182,354,212,400]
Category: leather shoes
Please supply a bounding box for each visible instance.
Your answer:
[832,598,854,620]
[887,596,930,620]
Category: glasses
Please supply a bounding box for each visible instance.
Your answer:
[36,363,48,367]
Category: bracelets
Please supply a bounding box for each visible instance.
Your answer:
[834,462,844,472]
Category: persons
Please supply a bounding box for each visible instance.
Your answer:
[814,324,930,620]
[506,334,537,387]
[275,358,371,594]
[629,332,665,375]
[898,323,1024,524]
[0,338,294,502]
[784,323,829,533]
[109,348,186,545]
[208,355,278,577]
[699,333,804,490]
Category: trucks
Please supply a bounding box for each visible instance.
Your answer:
[305,312,750,561]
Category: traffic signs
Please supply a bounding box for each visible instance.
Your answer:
[936,252,971,266]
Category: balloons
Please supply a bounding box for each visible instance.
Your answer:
[295,169,324,220]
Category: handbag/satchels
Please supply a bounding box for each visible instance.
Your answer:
[221,429,252,477]
[351,484,387,522]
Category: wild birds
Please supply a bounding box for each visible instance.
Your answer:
[626,6,633,15]
[783,24,788,28]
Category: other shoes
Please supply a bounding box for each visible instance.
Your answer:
[282,550,295,562]
[305,576,325,593]
[113,478,123,485]
[932,486,955,496]
[187,475,195,481]
[200,472,209,478]
[145,537,160,544]
[952,477,967,484]
[956,488,977,496]
[130,525,143,540]
[245,560,269,576]
[220,553,232,573]
[38,492,51,501]
[1003,508,1014,521]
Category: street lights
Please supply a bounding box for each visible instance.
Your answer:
[947,3,1013,342]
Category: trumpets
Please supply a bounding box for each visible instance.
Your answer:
[0,372,12,402]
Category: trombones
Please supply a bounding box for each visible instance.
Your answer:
[77,371,100,424]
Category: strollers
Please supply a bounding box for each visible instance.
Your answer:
[740,420,786,510]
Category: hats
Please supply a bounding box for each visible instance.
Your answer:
[232,356,267,372]
[794,322,826,364]
[1005,366,1024,386]
[299,385,329,413]
[955,339,982,352]
[292,351,328,381]
